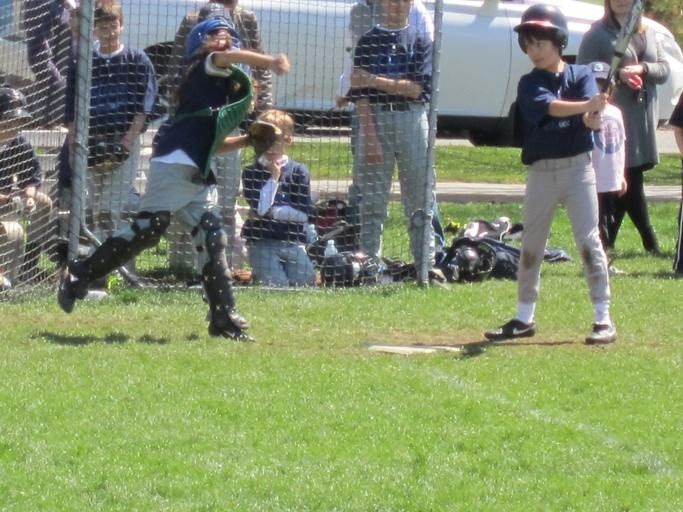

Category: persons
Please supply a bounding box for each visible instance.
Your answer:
[577,1,682,276]
[337,1,447,287]
[57,16,290,344]
[1,84,53,289]
[484,3,610,345]
[67,1,317,284]
[26,0,77,128]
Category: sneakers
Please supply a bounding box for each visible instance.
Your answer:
[608,265,628,277]
[484,319,536,340]
[208,318,255,343]
[58,259,76,314]
[585,324,618,345]
[416,268,449,291]
[17,266,55,288]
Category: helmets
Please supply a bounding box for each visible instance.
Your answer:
[185,19,242,63]
[442,237,497,281]
[513,4,568,54]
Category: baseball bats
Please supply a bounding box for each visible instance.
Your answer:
[594,1,645,114]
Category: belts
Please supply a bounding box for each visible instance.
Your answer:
[381,103,409,112]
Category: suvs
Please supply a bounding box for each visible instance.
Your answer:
[0,0,682,152]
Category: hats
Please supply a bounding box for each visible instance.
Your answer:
[0,87,33,119]
[587,61,615,86]
[198,2,232,24]
